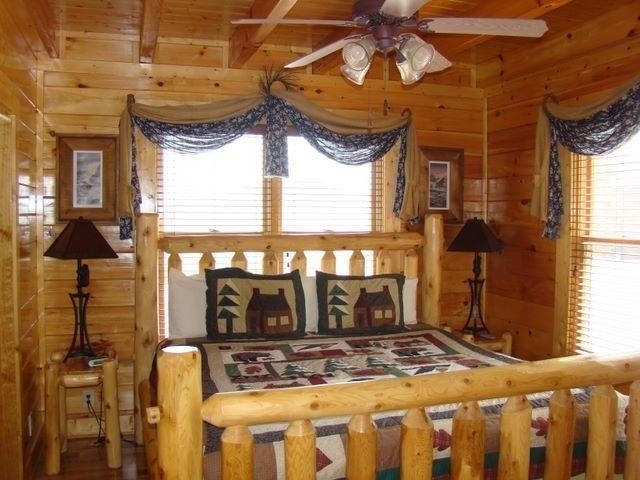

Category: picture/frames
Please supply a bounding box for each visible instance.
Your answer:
[56,132,120,226]
[418,145,464,224]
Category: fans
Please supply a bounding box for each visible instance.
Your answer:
[230,0,549,86]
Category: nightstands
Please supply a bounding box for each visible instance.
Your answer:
[45,351,122,474]
[445,322,512,356]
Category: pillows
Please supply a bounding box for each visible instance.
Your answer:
[315,271,411,334]
[204,266,307,340]
[168,266,306,338]
[301,270,418,333]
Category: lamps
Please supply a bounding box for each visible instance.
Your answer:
[43,216,118,363]
[339,32,436,86]
[447,216,503,337]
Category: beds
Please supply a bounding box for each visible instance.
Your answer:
[133,212,639,479]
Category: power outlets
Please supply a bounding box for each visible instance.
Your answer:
[82,390,94,410]
